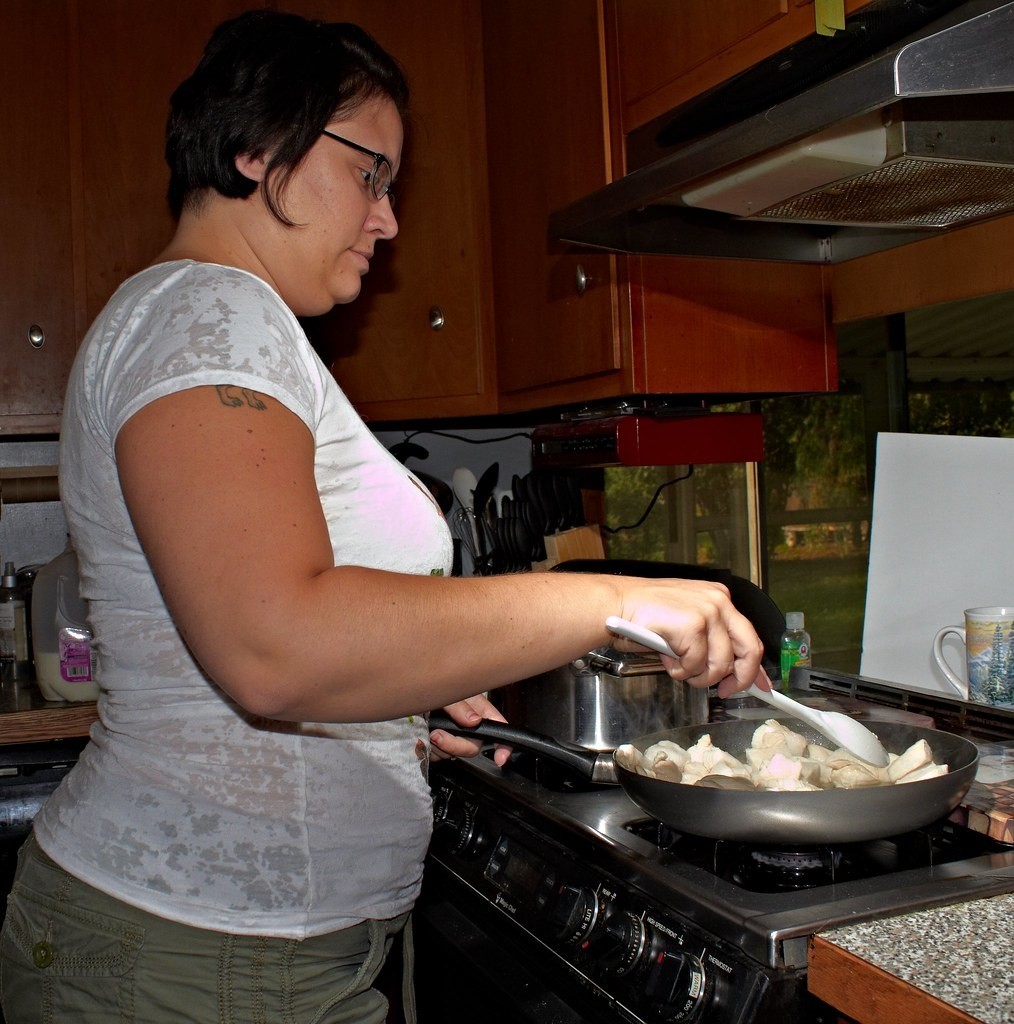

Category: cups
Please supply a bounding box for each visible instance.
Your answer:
[934,606,1014,710]
[0,660,35,715]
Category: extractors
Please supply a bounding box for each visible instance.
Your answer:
[546,0,1014,263]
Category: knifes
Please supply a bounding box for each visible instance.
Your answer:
[486,469,587,577]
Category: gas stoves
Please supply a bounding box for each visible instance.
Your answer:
[425,666,1012,1024]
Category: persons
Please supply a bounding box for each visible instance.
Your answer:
[0,11,771,1024]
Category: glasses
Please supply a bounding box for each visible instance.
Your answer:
[321,128,396,213]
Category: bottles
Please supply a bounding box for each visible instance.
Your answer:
[0,562,28,662]
[778,612,811,691]
[34,531,100,703]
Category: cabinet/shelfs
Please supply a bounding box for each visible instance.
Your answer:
[0,0,1014,437]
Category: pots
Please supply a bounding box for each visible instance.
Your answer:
[569,660,712,752]
[422,717,980,841]
